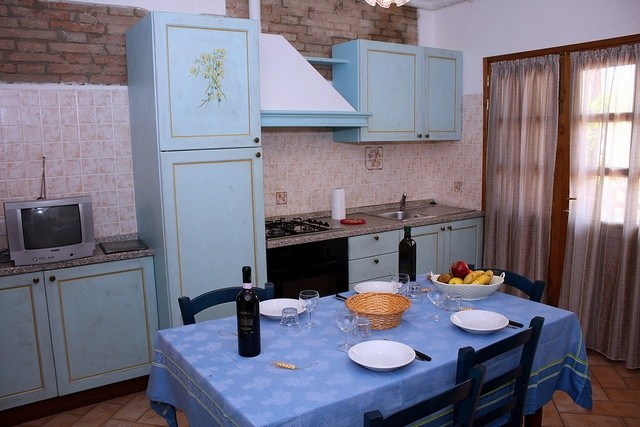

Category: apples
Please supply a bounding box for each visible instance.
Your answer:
[448,277,463,284]
[451,260,470,279]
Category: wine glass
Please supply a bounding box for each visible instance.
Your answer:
[336,309,354,353]
[299,290,319,329]
[427,284,448,321]
[393,273,410,295]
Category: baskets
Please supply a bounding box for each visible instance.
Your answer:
[346,292,411,330]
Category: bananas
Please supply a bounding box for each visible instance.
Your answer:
[464,269,485,284]
[471,269,493,285]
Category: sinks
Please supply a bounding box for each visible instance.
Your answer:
[414,205,465,216]
[375,211,422,222]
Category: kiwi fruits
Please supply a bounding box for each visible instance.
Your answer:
[437,273,451,283]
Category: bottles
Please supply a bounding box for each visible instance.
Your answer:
[399,226,416,284]
[236,266,261,357]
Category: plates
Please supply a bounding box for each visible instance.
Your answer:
[259,299,307,319]
[431,272,504,301]
[348,340,416,372]
[354,282,398,294]
[450,310,509,335]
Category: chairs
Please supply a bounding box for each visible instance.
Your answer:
[457,316,545,427]
[468,264,546,302]
[364,363,487,427]
[178,283,274,325]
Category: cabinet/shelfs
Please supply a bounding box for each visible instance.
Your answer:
[124,11,268,330]
[332,38,463,143]
[1,257,159,411]
[399,216,483,276]
[345,230,400,291]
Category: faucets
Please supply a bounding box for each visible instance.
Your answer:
[401,193,407,211]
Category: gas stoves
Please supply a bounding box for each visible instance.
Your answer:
[265,217,330,240]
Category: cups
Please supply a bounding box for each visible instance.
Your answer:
[351,317,371,343]
[445,294,462,317]
[279,307,301,336]
[407,281,421,300]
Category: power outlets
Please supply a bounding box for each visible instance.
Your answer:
[277,192,287,204]
